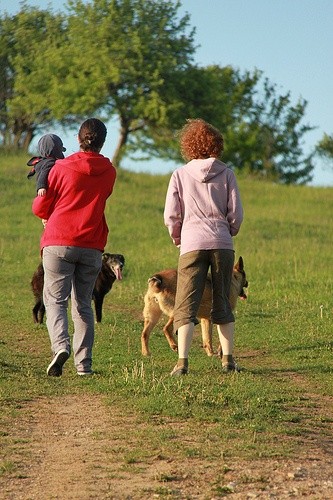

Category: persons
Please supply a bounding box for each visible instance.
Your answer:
[163,120,244,374]
[32,118,115,377]
[29,135,66,197]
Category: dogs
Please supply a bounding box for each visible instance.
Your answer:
[140,256,248,356]
[30,253,125,324]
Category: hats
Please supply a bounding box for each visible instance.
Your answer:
[37,134,66,158]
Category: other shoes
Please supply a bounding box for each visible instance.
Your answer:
[47,349,69,377]
[221,363,238,372]
[77,370,93,375]
[171,364,188,376]
